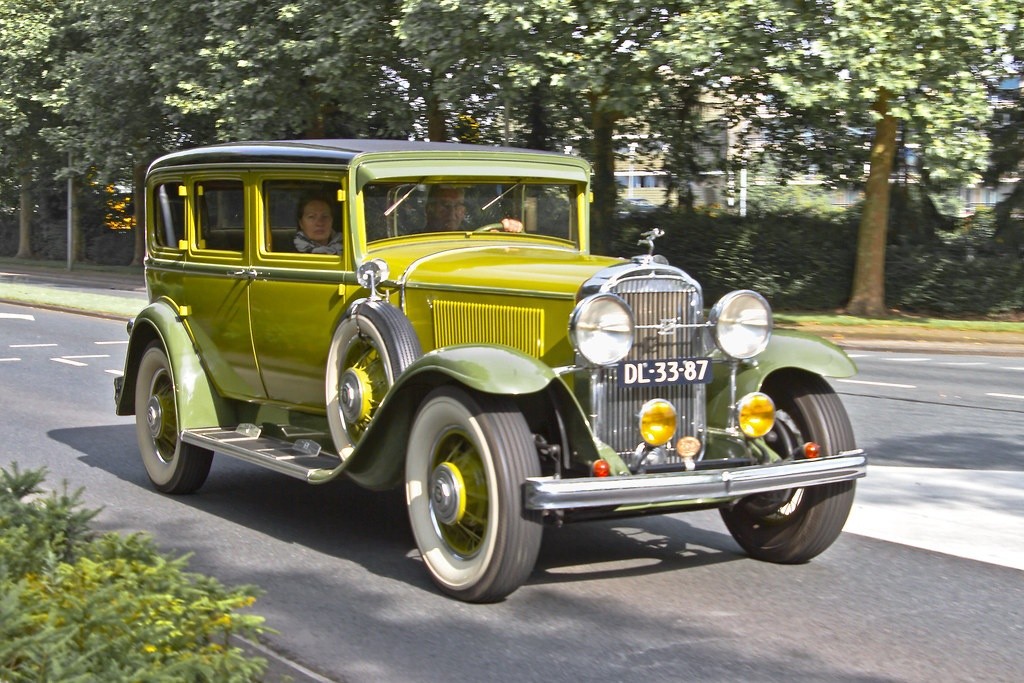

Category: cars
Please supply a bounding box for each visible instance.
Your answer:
[114,137,868,606]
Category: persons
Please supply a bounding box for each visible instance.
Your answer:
[289,193,347,257]
[421,184,524,234]
